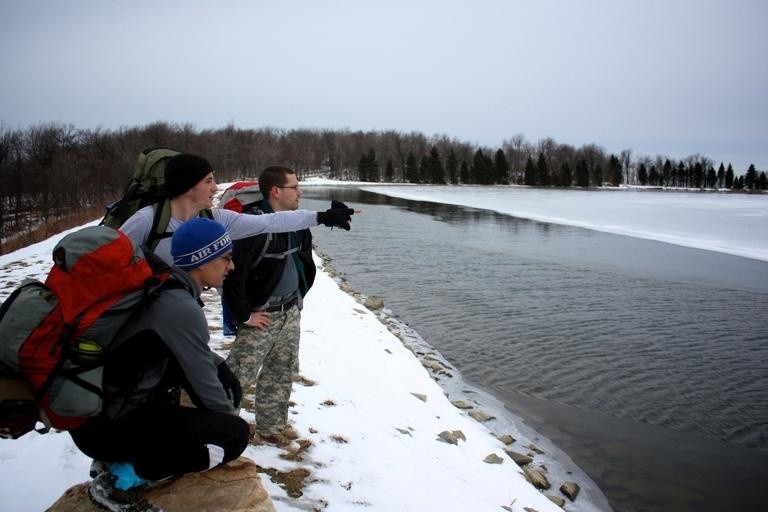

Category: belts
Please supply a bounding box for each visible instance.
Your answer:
[265,297,296,311]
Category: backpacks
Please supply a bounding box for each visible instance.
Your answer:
[218,183,264,213]
[0,226,170,439]
[99,149,182,252]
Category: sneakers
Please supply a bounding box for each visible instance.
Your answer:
[88,471,163,512]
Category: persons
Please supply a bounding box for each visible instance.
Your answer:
[65,218,251,511]
[211,166,318,448]
[118,153,363,269]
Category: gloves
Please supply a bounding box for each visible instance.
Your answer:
[217,361,242,408]
[318,200,353,231]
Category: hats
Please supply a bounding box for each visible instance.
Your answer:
[171,218,232,269]
[165,154,213,199]
